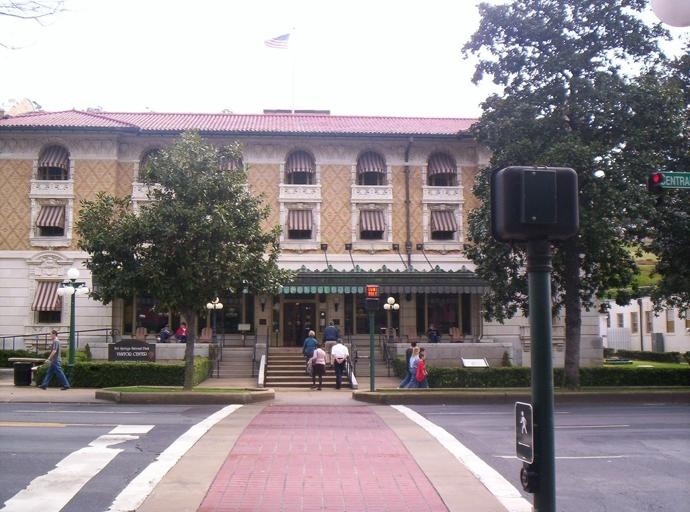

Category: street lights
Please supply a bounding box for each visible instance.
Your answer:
[383,296,400,336]
[56,267,89,364]
[206,296,224,343]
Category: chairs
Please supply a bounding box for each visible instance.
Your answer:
[385,327,399,343]
[131,327,148,343]
[199,327,212,343]
[449,327,464,343]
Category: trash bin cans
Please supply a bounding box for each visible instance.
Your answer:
[13,362,33,386]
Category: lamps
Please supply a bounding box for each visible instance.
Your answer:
[334,296,340,312]
[260,297,266,311]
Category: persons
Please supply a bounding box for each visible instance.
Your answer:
[331,337,349,389]
[38,330,70,390]
[157,324,171,343]
[449,321,461,343]
[398,339,430,388]
[302,330,318,376]
[310,344,328,391]
[425,323,442,343]
[322,320,338,368]
[175,322,187,343]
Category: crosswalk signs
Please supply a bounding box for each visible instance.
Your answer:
[514,402,536,465]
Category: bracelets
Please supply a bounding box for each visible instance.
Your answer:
[47,358,50,360]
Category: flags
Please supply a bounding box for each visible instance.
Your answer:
[263,32,291,50]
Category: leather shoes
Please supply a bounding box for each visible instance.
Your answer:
[39,385,46,390]
[61,387,69,390]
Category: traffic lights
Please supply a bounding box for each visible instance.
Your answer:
[647,170,664,195]
